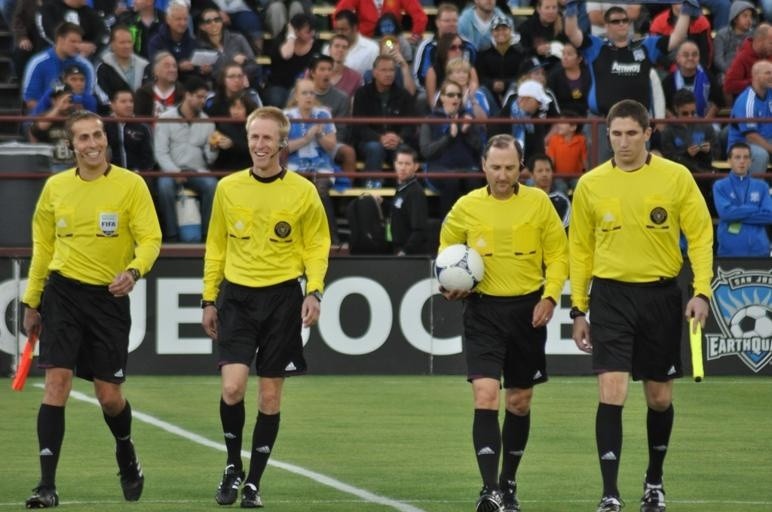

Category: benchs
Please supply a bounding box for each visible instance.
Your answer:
[0,1,535,236]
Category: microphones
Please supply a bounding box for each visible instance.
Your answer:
[511,163,525,188]
[270,138,289,158]
[63,138,85,158]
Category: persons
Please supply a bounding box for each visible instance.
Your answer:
[19,109,163,508]
[436,134,568,511]
[0,0,772,262]
[568,99,715,511]
[199,106,331,508]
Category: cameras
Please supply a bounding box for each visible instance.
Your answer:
[69,94,84,104]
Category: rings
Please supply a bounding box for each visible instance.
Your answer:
[546,317,549,321]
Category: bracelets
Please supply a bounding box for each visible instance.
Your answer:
[546,296,557,307]
[22,303,31,308]
[127,269,140,281]
[570,311,586,318]
[696,294,711,307]
[200,301,214,308]
[310,290,323,302]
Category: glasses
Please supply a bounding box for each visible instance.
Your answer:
[683,111,694,115]
[447,93,462,97]
[609,19,628,24]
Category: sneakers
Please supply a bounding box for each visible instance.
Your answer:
[116,440,143,502]
[640,482,666,512]
[598,495,621,512]
[476,480,521,512]
[241,483,262,506]
[26,485,58,508]
[215,462,245,504]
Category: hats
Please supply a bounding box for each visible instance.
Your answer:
[491,17,511,29]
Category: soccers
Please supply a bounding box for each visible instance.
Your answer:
[434,244,484,292]
